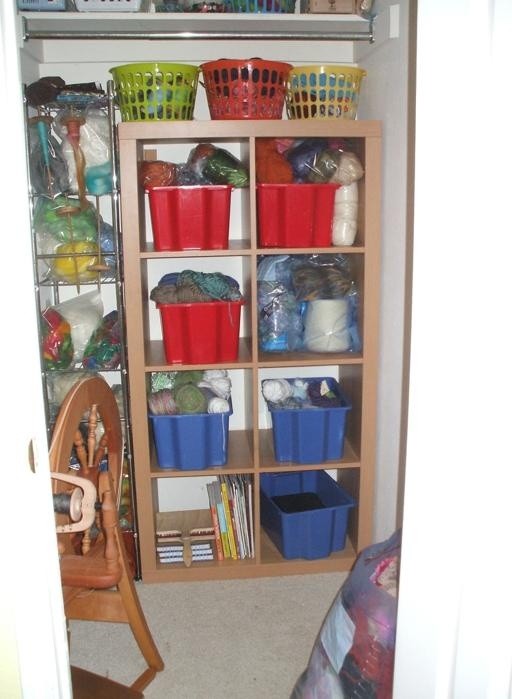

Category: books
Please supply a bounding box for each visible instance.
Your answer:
[156,473,255,567]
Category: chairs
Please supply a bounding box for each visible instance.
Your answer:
[49,375,165,699]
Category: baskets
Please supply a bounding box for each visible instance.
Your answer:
[282,64,368,120]
[16,0,300,13]
[107,62,200,122]
[197,58,294,122]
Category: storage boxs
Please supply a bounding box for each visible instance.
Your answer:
[253,182,344,248]
[262,376,352,464]
[258,469,355,559]
[148,397,233,471]
[144,182,235,250]
[155,298,248,363]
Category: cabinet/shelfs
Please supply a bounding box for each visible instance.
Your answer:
[119,118,379,582]
